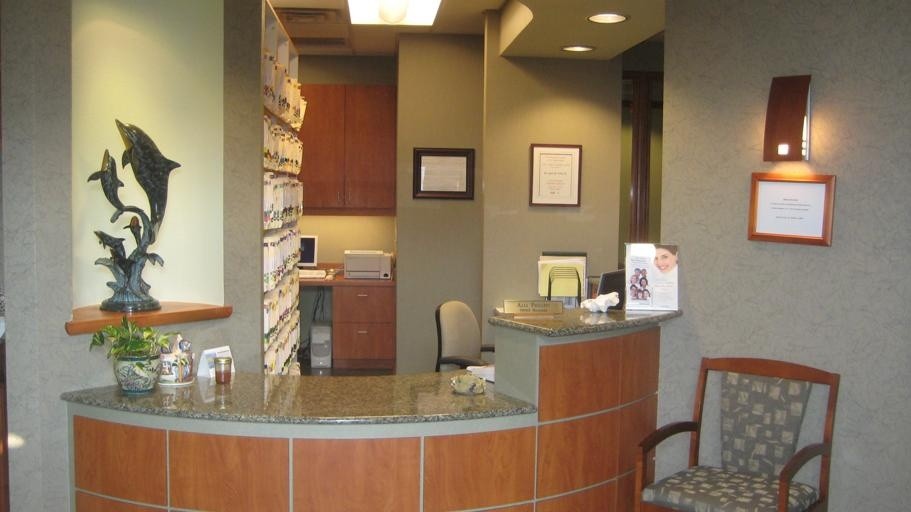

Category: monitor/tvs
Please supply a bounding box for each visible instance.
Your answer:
[596,268,625,309]
[297,235,318,267]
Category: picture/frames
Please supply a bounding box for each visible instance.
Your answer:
[411,145,475,201]
[529,142,583,209]
[746,170,837,248]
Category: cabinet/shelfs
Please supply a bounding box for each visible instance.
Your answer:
[299,82,397,218]
[260,0,302,378]
[299,270,396,372]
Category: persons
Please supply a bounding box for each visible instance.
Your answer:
[629,268,651,300]
[653,244,678,272]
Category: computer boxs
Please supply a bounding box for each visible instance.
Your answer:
[310,320,332,368]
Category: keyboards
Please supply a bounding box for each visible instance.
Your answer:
[299,270,326,278]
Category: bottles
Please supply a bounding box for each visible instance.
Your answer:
[214,358,232,385]
[214,385,231,413]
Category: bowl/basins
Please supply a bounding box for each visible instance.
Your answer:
[449,375,487,393]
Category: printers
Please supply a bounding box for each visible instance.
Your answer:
[344,250,391,279]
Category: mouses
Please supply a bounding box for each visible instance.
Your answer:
[325,274,334,281]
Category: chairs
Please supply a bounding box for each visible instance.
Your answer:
[434,299,494,374]
[633,357,842,512]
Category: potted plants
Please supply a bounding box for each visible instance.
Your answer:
[88,315,181,396]
